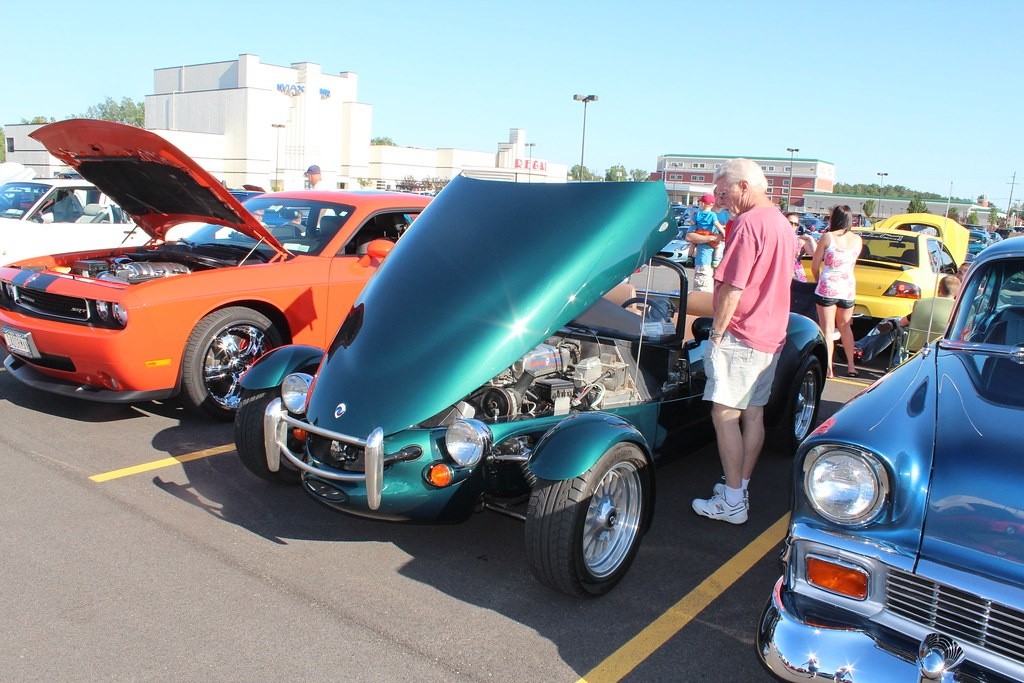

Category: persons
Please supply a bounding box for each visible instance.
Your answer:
[685,186,731,292]
[836,264,984,370]
[1021,231,1024,235]
[687,195,725,267]
[811,205,863,379]
[690,158,796,525]
[255,211,269,229]
[834,667,853,683]
[283,211,302,224]
[864,216,872,226]
[786,213,816,282]
[799,659,819,679]
[762,626,775,655]
[307,165,335,232]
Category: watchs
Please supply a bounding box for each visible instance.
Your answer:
[709,329,722,337]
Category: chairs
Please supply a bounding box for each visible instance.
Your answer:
[859,244,870,258]
[604,284,643,316]
[968,305,1024,406]
[668,291,715,346]
[901,249,916,266]
[320,213,349,237]
[75,204,113,223]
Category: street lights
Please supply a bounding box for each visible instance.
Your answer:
[271,124,286,191]
[524,143,538,184]
[786,148,800,211]
[876,172,888,219]
[572,94,599,182]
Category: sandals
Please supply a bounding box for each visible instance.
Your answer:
[849,370,859,378]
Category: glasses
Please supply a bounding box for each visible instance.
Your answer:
[789,221,800,228]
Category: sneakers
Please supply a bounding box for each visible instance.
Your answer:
[692,490,748,523]
[686,258,693,266]
[711,261,720,268]
[713,476,750,511]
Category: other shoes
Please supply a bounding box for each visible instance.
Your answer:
[878,320,896,335]
[835,343,863,364]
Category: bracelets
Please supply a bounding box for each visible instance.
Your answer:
[710,236,714,242]
[809,237,813,243]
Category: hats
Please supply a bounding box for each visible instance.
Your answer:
[306,165,320,174]
[697,195,716,206]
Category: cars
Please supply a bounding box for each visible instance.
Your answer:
[0,171,310,274]
[234,170,828,598]
[650,201,1024,362]
[0,117,439,422]
[754,238,1024,683]
